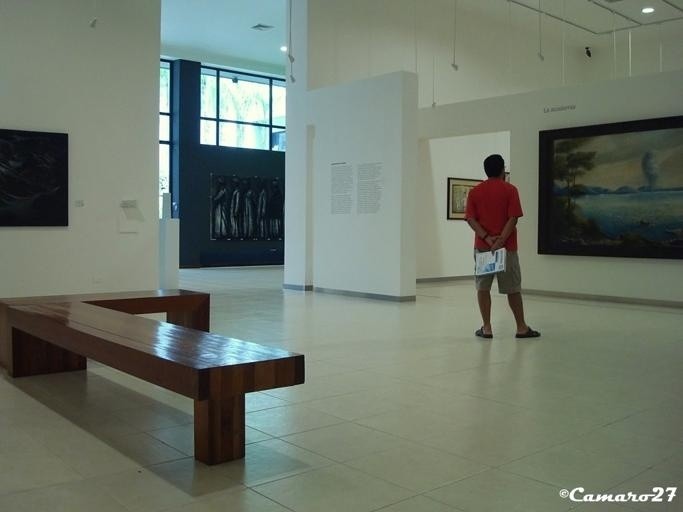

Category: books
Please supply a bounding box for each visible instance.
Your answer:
[474,247,507,275]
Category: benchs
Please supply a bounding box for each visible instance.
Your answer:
[0,288,305,465]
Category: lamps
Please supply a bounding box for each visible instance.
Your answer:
[287,0,545,108]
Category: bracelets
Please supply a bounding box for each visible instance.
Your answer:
[481,233,489,240]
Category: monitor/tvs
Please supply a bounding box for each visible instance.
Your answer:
[0,127,70,227]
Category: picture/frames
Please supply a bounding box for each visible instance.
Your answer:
[504,172,510,183]
[537,116,683,260]
[446,177,484,220]
[210,174,284,242]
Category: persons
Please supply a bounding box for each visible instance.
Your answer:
[211,173,285,240]
[462,153,541,340]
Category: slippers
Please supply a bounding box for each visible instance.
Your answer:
[474,326,493,338]
[514,327,540,338]
